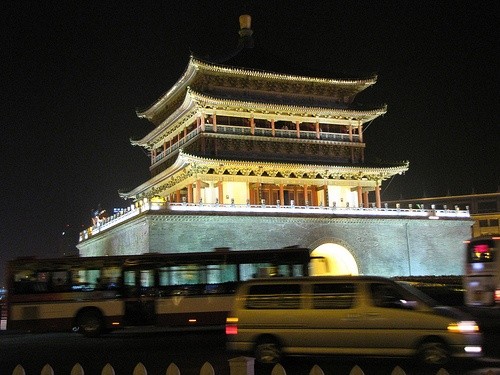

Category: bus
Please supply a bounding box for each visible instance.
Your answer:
[0,246,311,337]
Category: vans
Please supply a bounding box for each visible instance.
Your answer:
[225,275,486,375]
[463,232,500,321]
